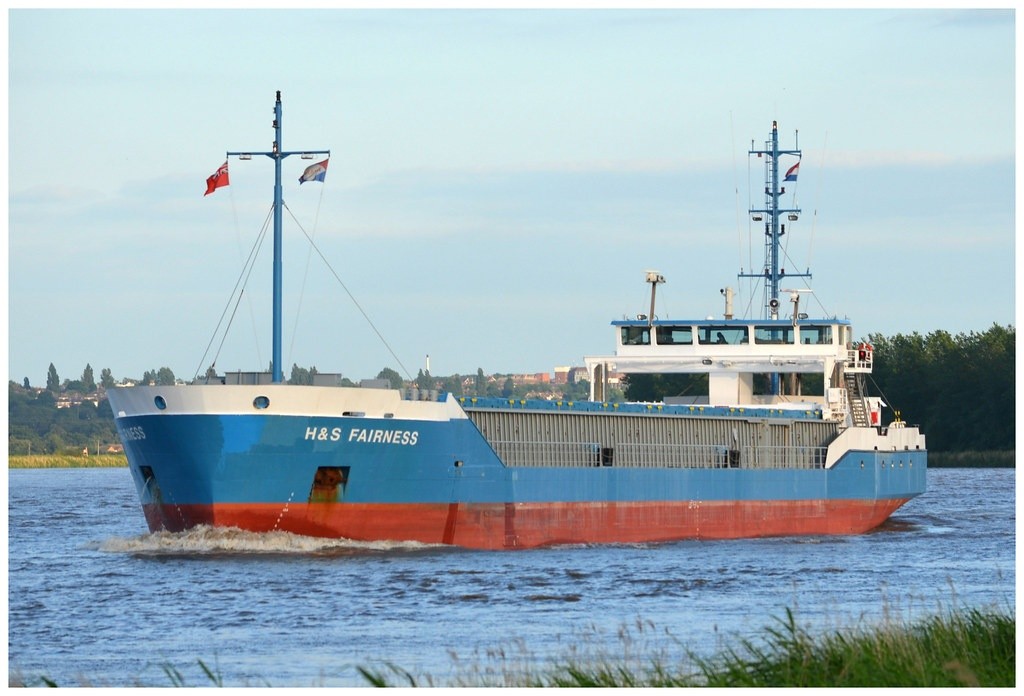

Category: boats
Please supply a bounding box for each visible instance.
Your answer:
[101,89,926,549]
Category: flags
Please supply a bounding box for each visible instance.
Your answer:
[204,159,228,195]
[783,162,800,182]
[300,159,329,184]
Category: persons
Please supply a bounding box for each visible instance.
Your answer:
[717,332,727,344]
